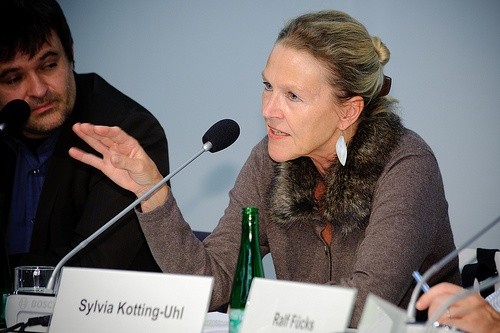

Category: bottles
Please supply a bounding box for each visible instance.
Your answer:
[229,206,265,333]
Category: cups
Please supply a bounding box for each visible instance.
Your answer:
[14,265,64,298]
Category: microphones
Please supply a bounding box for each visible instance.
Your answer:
[0,99,31,136]
[5,119,240,332]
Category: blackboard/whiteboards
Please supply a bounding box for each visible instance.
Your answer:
[55,0,500,252]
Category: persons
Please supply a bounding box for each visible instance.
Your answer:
[68,9,464,330]
[0,0,171,333]
[415,280,500,333]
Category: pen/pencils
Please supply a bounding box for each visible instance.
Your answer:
[412,271,429,292]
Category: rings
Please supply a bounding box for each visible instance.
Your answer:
[446,307,453,320]
